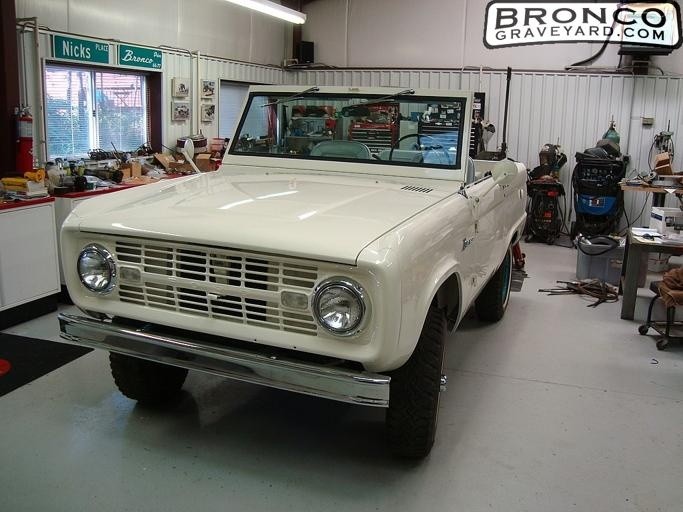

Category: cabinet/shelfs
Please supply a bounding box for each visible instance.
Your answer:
[122,152,131,162]
[58,158,85,175]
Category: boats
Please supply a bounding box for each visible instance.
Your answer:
[296,41,314,64]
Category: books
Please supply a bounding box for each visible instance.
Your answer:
[115,160,141,179]
[194,153,211,173]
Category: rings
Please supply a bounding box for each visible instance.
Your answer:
[112,152,120,165]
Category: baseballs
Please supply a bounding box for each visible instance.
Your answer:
[15,104,32,173]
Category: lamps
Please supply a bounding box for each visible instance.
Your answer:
[308,138,476,190]
[638,281,683,351]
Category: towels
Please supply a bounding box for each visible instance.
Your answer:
[576,236,614,281]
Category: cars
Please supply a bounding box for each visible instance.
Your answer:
[87,181,96,190]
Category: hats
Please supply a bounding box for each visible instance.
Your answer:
[58,85,528,458]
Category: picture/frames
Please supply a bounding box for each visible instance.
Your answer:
[616,227,682,319]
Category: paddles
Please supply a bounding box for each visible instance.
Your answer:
[0,330,96,400]
